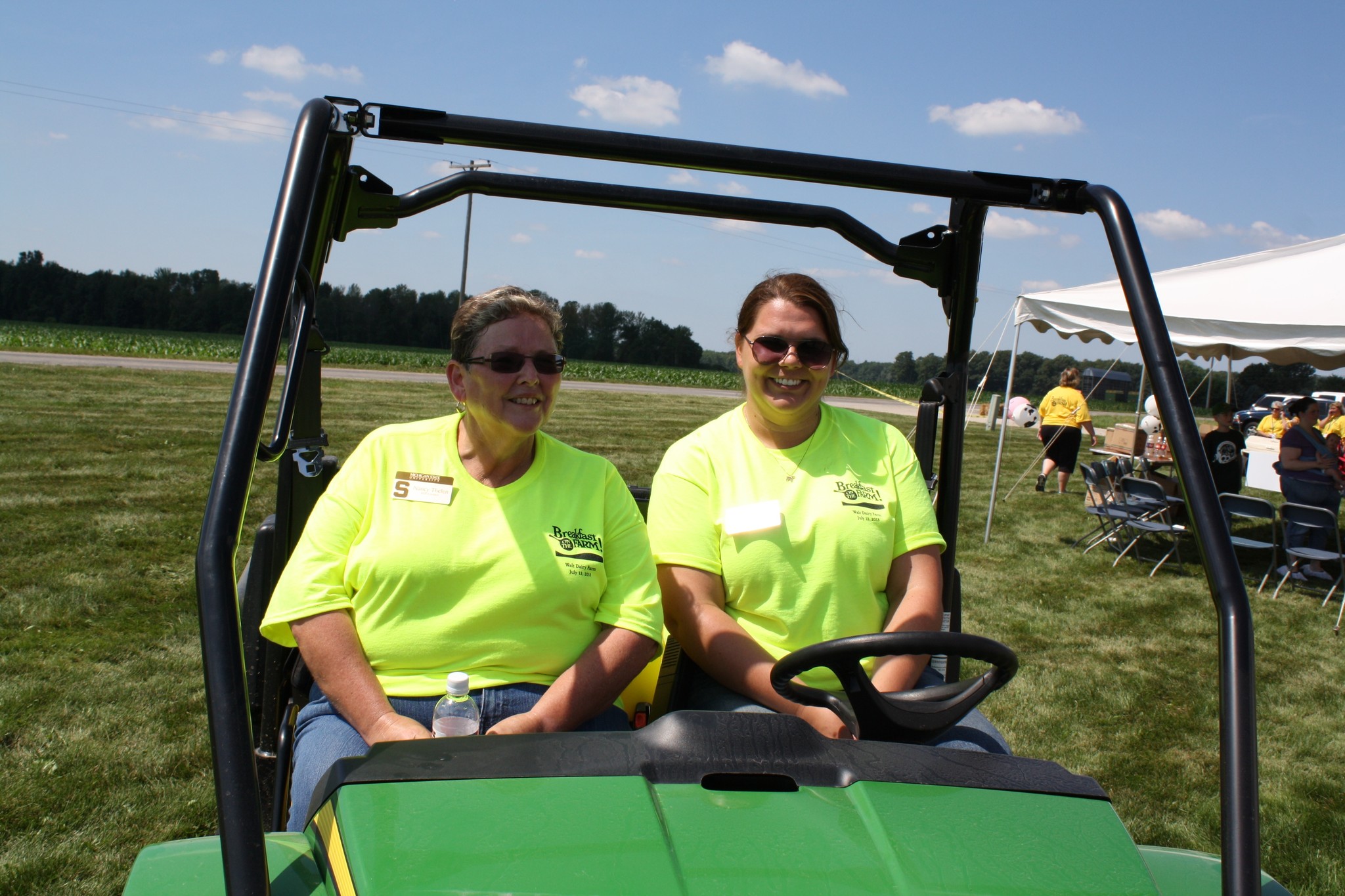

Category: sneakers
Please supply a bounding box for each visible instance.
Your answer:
[1035,473,1046,492]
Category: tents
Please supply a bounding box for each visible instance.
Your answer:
[983,234,1344,634]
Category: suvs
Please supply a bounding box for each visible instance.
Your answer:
[1232,391,1345,439]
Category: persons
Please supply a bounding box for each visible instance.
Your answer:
[647,273,1012,756]
[1202,402,1246,536]
[1273,396,1341,583]
[260,283,664,835]
[1319,401,1345,496]
[1256,399,1291,439]
[1316,432,1345,490]
[1285,415,1321,430]
[1036,367,1098,494]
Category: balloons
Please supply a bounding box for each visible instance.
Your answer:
[1145,395,1160,416]
[1012,403,1039,427]
[1140,415,1162,434]
[1007,396,1031,421]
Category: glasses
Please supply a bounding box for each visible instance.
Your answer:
[1271,408,1280,411]
[463,352,567,375]
[739,330,836,370]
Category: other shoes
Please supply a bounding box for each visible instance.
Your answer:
[1299,564,1334,581]
[1278,565,1307,580]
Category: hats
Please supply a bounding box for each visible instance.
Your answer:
[1213,402,1234,415]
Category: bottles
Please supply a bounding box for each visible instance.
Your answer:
[1145,435,1154,453]
[1155,437,1163,454]
[1161,437,1168,457]
[433,671,479,736]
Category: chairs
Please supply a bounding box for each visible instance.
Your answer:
[1077,452,1345,636]
